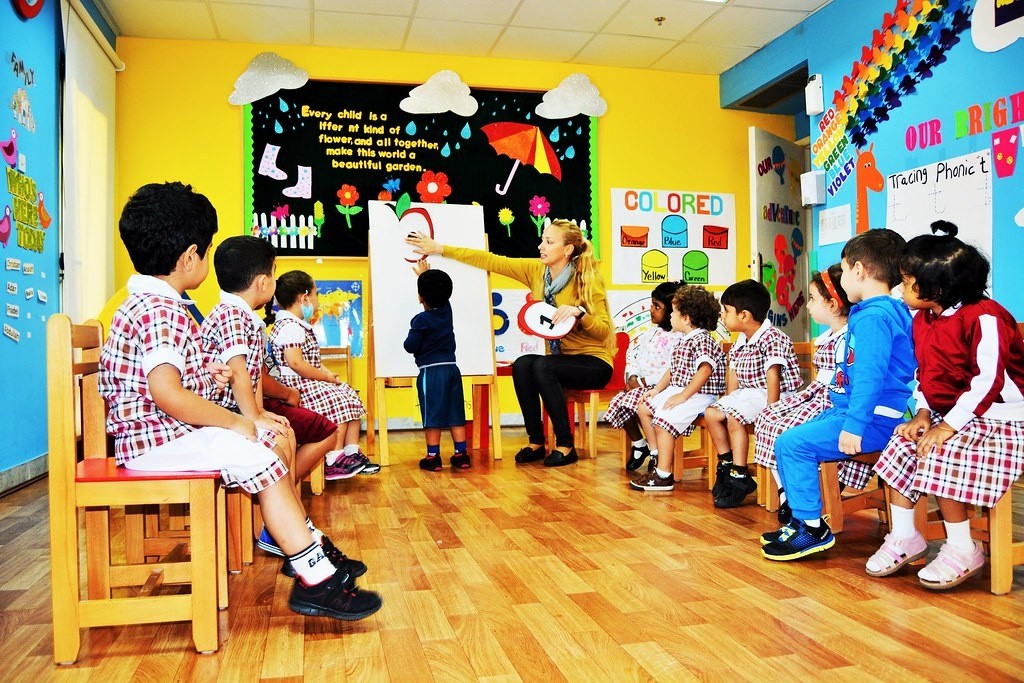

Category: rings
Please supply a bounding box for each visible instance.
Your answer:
[420,236,422,239]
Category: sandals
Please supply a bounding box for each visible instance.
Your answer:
[919,541,984,589]
[866,530,927,576]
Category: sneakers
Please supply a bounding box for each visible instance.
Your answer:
[627,443,651,471]
[761,516,836,562]
[631,466,674,491]
[288,567,382,620]
[760,516,794,545]
[712,463,733,497]
[714,469,757,507]
[346,449,381,475]
[648,455,659,472]
[280,533,367,579]
[324,452,366,480]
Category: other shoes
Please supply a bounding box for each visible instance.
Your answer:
[450,454,470,467]
[544,446,578,467]
[257,527,284,555]
[515,446,546,463]
[419,456,442,471]
[778,486,793,524]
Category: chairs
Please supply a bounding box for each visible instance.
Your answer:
[46,313,1024,666]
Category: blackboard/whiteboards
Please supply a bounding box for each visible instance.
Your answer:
[367,199,496,385]
[242,78,600,261]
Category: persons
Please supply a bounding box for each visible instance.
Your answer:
[629,285,728,491]
[195,235,338,556]
[403,258,471,472]
[603,279,687,475]
[262,270,379,479]
[756,264,859,521]
[95,180,382,622]
[704,278,805,509]
[866,218,1024,589]
[405,217,617,468]
[760,227,920,561]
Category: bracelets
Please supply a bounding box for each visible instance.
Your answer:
[936,424,954,432]
